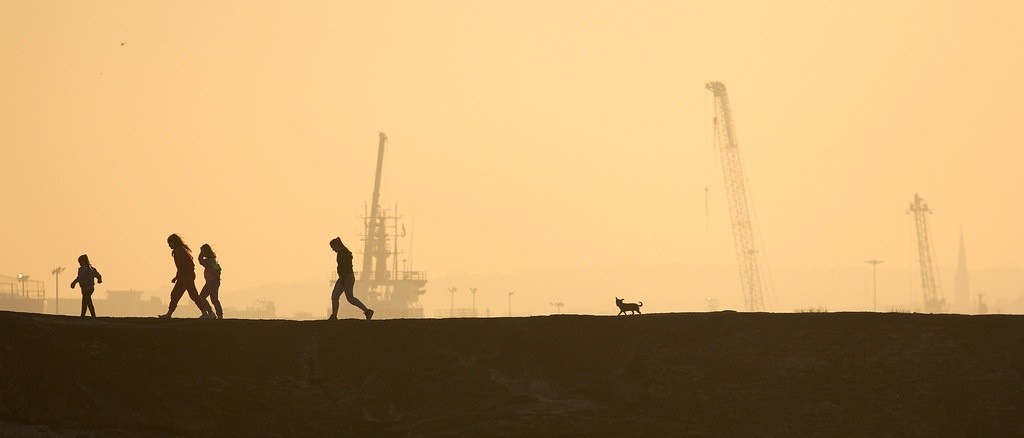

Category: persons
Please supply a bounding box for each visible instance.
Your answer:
[70,254,102,318]
[328,236,374,320]
[158,234,210,320]
[198,243,223,319]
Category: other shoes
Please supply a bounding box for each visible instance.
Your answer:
[158,314,171,319]
[328,314,338,320]
[216,313,223,319]
[199,312,216,319]
[364,309,374,320]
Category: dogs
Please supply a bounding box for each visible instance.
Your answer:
[615,296,642,316]
